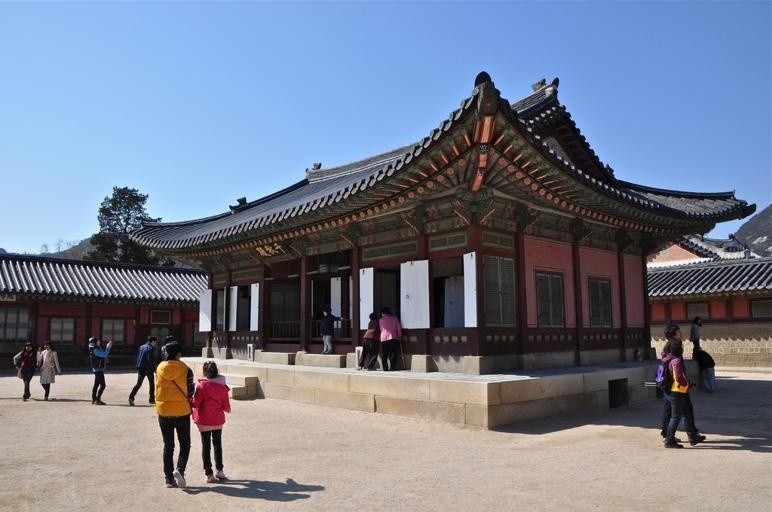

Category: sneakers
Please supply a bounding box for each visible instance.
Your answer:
[45,396,48,400]
[23,393,30,401]
[691,436,705,445]
[357,366,399,370]
[92,399,105,405]
[215,469,226,478]
[129,397,134,406]
[149,399,155,404]
[322,350,332,354]
[661,431,683,448]
[206,474,217,483]
[167,480,177,487]
[173,468,186,487]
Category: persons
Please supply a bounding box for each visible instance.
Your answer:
[655,315,716,446]
[379,307,402,371]
[89,335,112,405]
[128,335,157,406]
[160,336,175,361]
[36,340,61,401]
[357,313,381,371]
[319,307,344,354]
[190,361,231,483]
[154,341,195,488]
[13,343,36,402]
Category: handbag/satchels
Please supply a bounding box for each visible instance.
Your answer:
[17,368,23,379]
[656,358,674,393]
